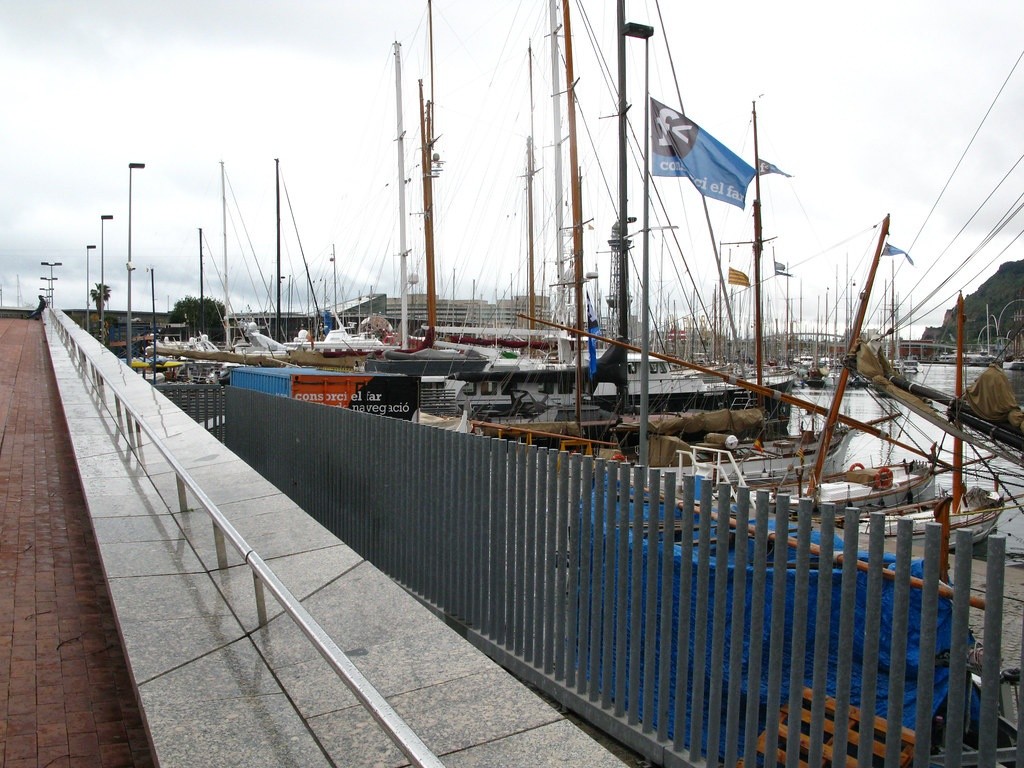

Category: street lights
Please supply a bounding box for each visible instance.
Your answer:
[125,162,147,369]
[100,214,115,344]
[40,288,54,306]
[86,245,97,333]
[41,261,62,308]
[40,276,58,305]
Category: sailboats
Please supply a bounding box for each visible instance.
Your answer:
[146,0,1024,471]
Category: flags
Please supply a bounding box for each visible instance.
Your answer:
[776,270,792,277]
[586,291,602,380]
[758,158,793,177]
[775,262,785,270]
[323,312,333,335]
[650,98,757,211]
[882,243,915,266]
[729,267,750,286]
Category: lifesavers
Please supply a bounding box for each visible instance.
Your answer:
[849,462,865,472]
[873,467,893,490]
[383,334,396,345]
[610,453,625,465]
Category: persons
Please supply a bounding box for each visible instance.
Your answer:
[26,295,46,318]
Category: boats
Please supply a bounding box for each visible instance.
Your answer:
[859,472,1007,553]
[748,459,938,518]
